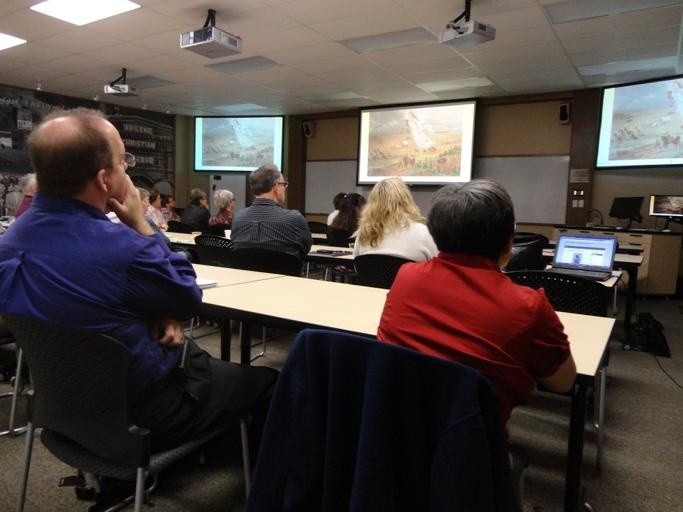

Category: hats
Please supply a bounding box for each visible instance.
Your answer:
[188,186,207,201]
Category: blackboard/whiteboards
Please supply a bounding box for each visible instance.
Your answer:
[305,153,570,226]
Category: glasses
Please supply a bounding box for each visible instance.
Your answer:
[93,152,136,175]
[228,197,236,202]
[269,180,289,190]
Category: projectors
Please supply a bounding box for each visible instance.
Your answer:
[439,21,497,49]
[179,25,244,58]
[103,84,139,97]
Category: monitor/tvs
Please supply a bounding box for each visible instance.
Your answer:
[649,193,683,231]
[608,196,644,230]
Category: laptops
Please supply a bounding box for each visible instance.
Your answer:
[544,235,618,281]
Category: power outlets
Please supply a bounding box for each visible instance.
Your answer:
[572,199,584,207]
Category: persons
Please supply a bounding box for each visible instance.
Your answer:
[230,162,313,256]
[353,177,439,275]
[133,187,193,261]
[149,190,169,232]
[180,187,212,234]
[161,194,181,225]
[331,193,368,246]
[328,192,347,230]
[377,178,577,422]
[0,108,280,464]
[14,173,37,219]
[208,190,236,228]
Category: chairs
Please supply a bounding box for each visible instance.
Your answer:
[0,311,252,512]
[186,261,285,364]
[296,328,519,512]
[504,271,609,317]
[352,253,418,290]
[0,330,35,436]
[222,248,300,362]
[194,235,235,266]
[306,219,349,248]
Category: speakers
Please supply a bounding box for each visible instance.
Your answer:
[302,121,315,137]
[558,103,568,122]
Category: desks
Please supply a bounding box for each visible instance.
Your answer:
[541,240,646,350]
[306,245,354,284]
[200,275,616,512]
[162,230,200,245]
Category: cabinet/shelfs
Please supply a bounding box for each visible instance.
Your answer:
[209,174,245,216]
[553,225,683,295]
